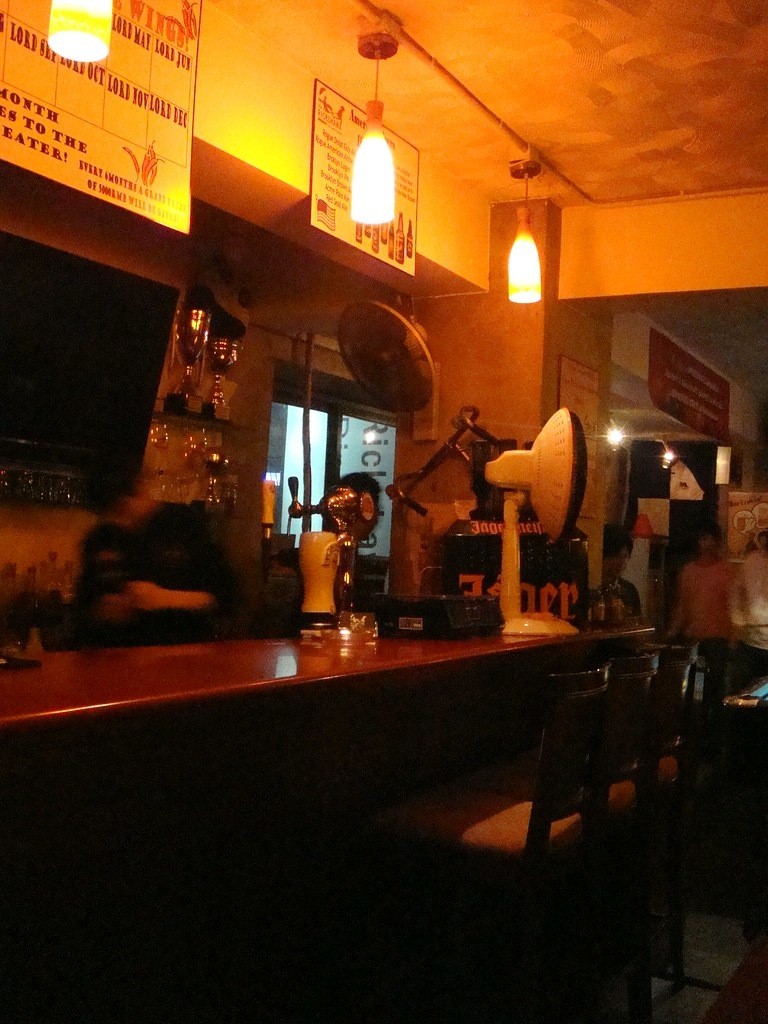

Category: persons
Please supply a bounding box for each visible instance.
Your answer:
[665,519,752,710]
[729,531,767,684]
[70,463,246,649]
[321,472,382,580]
[602,535,641,614]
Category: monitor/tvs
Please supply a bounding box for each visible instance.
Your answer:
[0,233,181,512]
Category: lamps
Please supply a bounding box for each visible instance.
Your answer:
[48,0,113,62]
[508,161,542,304]
[350,35,397,226]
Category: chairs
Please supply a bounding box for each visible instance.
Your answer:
[462,651,661,1024]
[366,659,617,1024]
[523,636,701,1024]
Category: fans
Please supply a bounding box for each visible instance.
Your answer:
[486,408,587,637]
[339,300,435,415]
